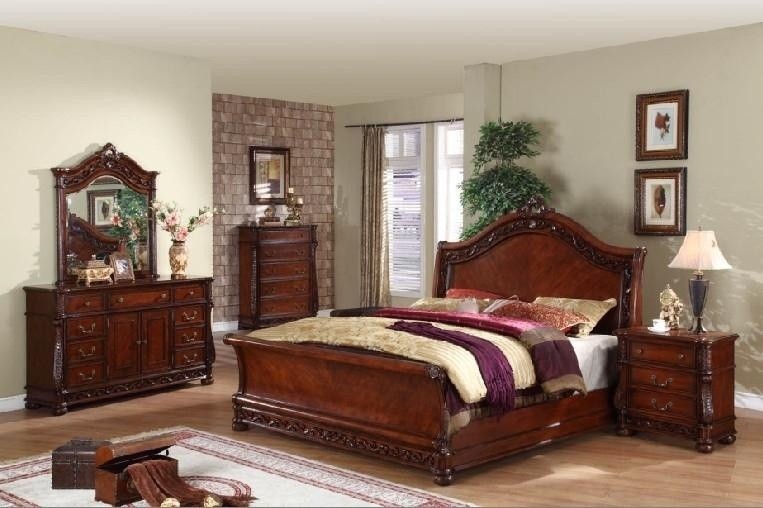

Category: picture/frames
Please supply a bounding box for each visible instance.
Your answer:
[634,89,688,161]
[633,168,687,237]
[86,189,121,232]
[90,177,121,185]
[249,146,292,206]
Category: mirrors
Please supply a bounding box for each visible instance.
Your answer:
[51,142,160,287]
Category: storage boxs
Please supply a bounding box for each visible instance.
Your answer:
[94,434,177,507]
[52,435,112,491]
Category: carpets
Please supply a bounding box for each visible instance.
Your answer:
[0,424,487,508]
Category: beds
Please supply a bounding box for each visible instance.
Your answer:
[223,293,622,487]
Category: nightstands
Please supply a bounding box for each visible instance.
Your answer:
[618,326,738,453]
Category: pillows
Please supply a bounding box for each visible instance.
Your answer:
[412,296,494,315]
[536,294,617,336]
[440,286,504,317]
[482,299,584,338]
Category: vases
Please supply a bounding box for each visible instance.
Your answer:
[168,239,190,279]
[134,239,151,272]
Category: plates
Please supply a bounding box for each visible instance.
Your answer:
[647,327,670,333]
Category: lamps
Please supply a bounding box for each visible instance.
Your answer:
[665,230,733,337]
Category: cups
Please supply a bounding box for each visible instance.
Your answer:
[653,319,665,327]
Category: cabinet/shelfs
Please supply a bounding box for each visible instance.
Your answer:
[243,225,320,327]
[25,277,212,412]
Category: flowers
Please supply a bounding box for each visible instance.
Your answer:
[112,187,148,267]
[154,200,217,240]
